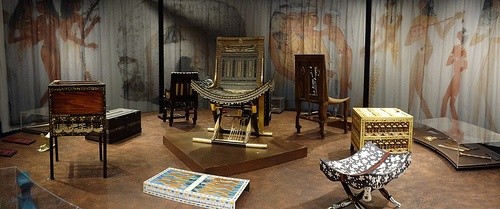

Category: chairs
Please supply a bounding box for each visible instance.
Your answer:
[295,54,351,139]
[163,71,199,126]
